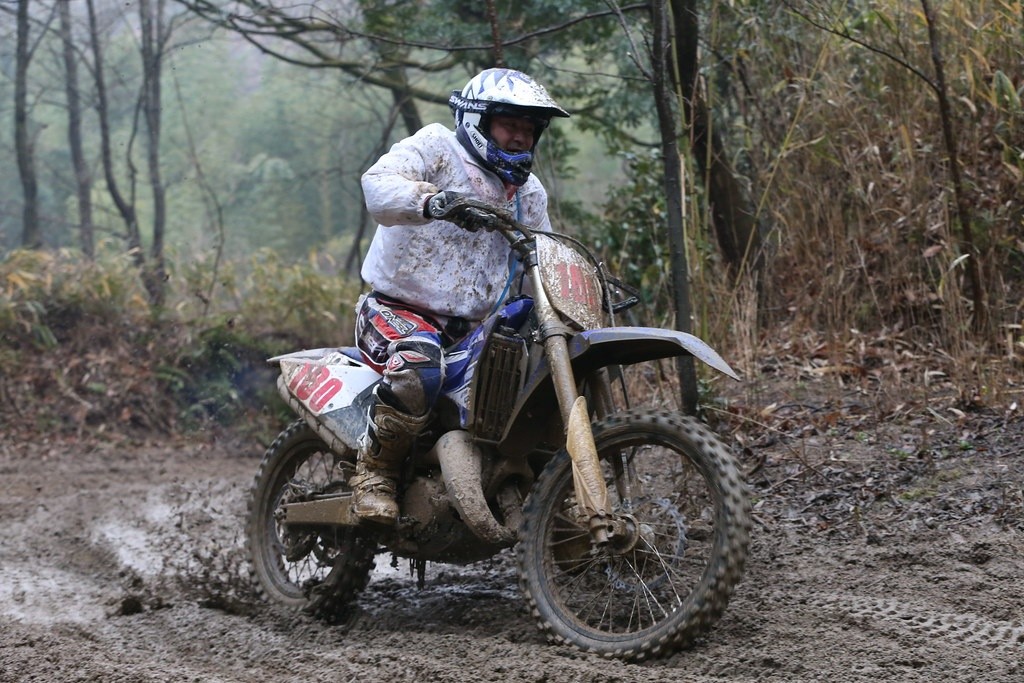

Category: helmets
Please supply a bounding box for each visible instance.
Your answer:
[448,68,571,186]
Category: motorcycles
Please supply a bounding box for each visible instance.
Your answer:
[244,198,750,657]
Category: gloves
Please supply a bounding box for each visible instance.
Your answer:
[428,190,480,233]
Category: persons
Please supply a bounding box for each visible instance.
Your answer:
[348,66,571,519]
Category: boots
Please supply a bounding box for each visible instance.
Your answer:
[347,384,431,525]
